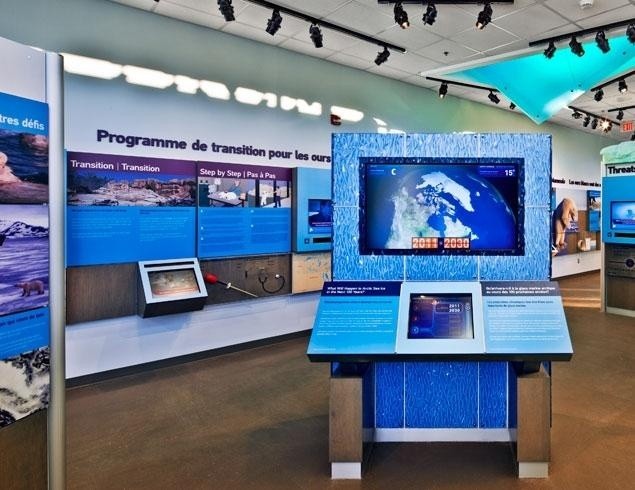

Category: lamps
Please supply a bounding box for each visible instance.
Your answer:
[530,18,635,64]
[379,1,514,31]
[425,70,635,133]
[212,1,406,66]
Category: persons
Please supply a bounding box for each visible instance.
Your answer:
[270,185,282,208]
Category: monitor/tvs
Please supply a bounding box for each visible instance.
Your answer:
[611,200,635,230]
[358,156,524,255]
[148,267,201,297]
[395,281,486,354]
